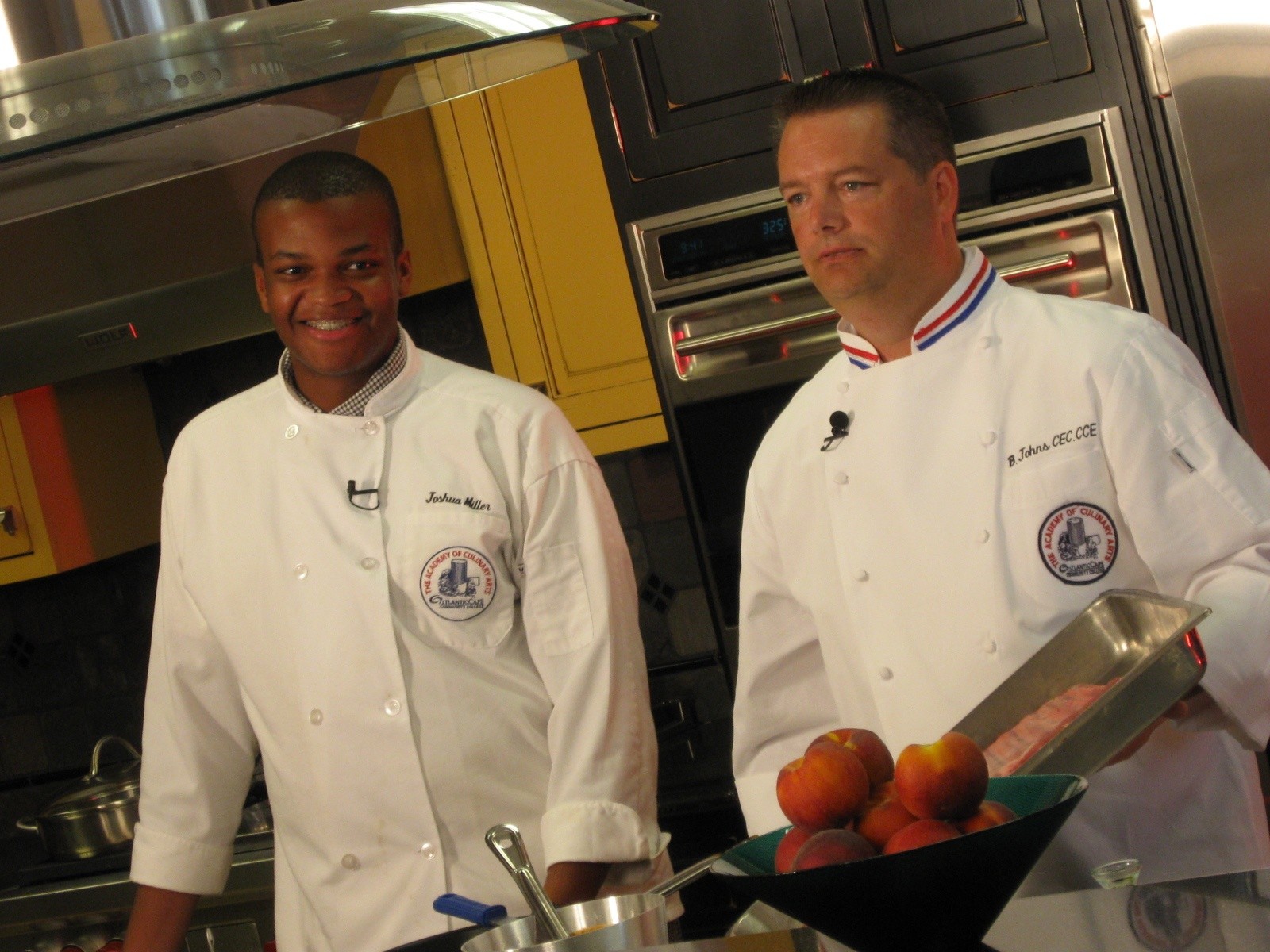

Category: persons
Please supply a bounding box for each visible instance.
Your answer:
[119,148,690,951]
[725,67,1270,952]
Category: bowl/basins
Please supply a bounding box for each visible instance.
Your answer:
[709,774,1091,951]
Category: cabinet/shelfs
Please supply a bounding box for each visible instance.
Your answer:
[0,369,164,584]
[430,63,670,458]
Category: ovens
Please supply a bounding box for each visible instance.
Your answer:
[623,107,1171,669]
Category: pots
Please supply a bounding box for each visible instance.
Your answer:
[460,834,759,952]
[15,734,140,861]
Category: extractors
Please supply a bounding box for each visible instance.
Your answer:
[0,0,469,389]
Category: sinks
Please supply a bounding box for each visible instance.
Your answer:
[225,797,275,854]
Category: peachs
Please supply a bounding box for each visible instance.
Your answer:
[773,726,1024,871]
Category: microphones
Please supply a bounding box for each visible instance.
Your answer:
[829,412,848,436]
[347,480,356,500]
